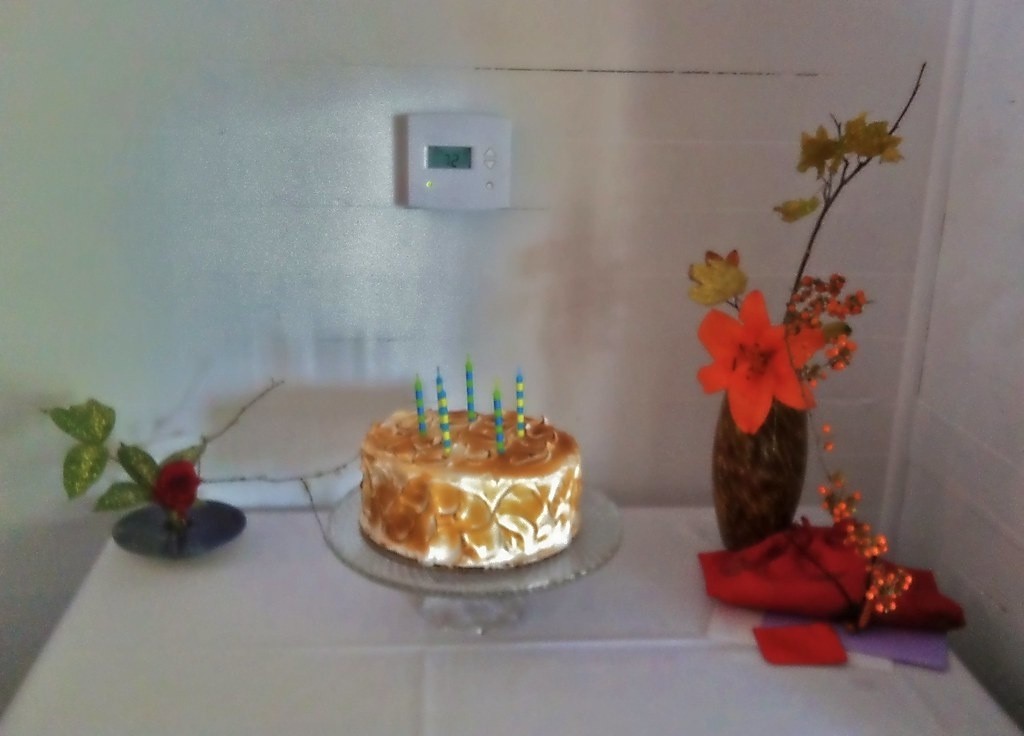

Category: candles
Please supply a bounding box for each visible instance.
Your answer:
[464,355,474,422]
[514,375,526,436]
[415,372,428,438]
[436,365,448,416]
[492,380,506,450]
[439,388,453,454]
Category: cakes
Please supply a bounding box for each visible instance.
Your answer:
[359,407,583,569]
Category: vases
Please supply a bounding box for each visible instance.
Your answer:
[710,386,809,552]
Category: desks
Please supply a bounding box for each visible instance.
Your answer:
[0,504,1024,736]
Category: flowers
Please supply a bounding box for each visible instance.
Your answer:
[686,60,932,634]
[37,374,288,522]
